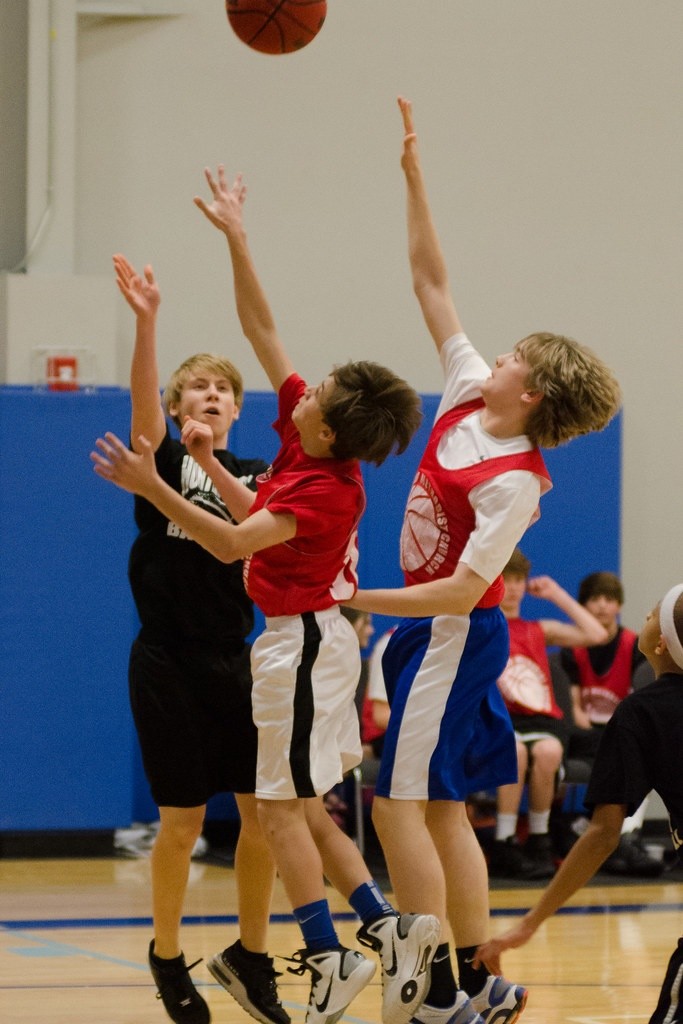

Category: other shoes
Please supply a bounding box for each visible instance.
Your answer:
[493,832,557,880]
[601,828,662,877]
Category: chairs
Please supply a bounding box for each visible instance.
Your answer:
[343,647,660,868]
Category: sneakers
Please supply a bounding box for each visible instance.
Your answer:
[207,939,291,1024]
[406,990,486,1024]
[275,944,377,1024]
[469,976,528,1024]
[148,938,210,1024]
[356,911,440,1024]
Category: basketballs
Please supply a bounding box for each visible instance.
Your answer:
[224,0,328,55]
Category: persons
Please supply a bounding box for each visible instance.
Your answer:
[91,164,440,1024]
[323,607,401,829]
[341,96,618,1024]
[493,547,610,882]
[561,572,662,880]
[471,587,683,1024]
[111,254,296,1024]
